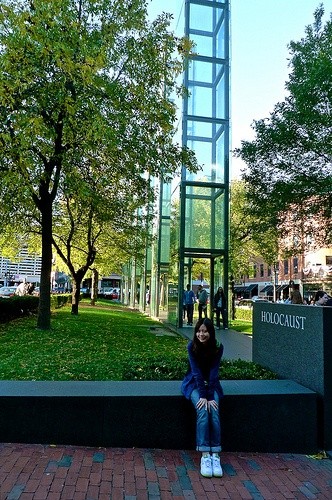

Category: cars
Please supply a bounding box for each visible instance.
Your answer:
[0,287,68,297]
[239,299,269,306]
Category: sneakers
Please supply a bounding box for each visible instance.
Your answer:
[211,454,223,477]
[201,455,213,478]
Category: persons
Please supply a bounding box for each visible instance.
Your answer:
[285,285,332,306]
[183,284,208,326]
[214,287,228,329]
[16,278,32,297]
[181,318,224,476]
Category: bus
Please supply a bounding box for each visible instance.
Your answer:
[81,276,120,299]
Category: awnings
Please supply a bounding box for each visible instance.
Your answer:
[260,285,288,292]
[234,284,258,292]
